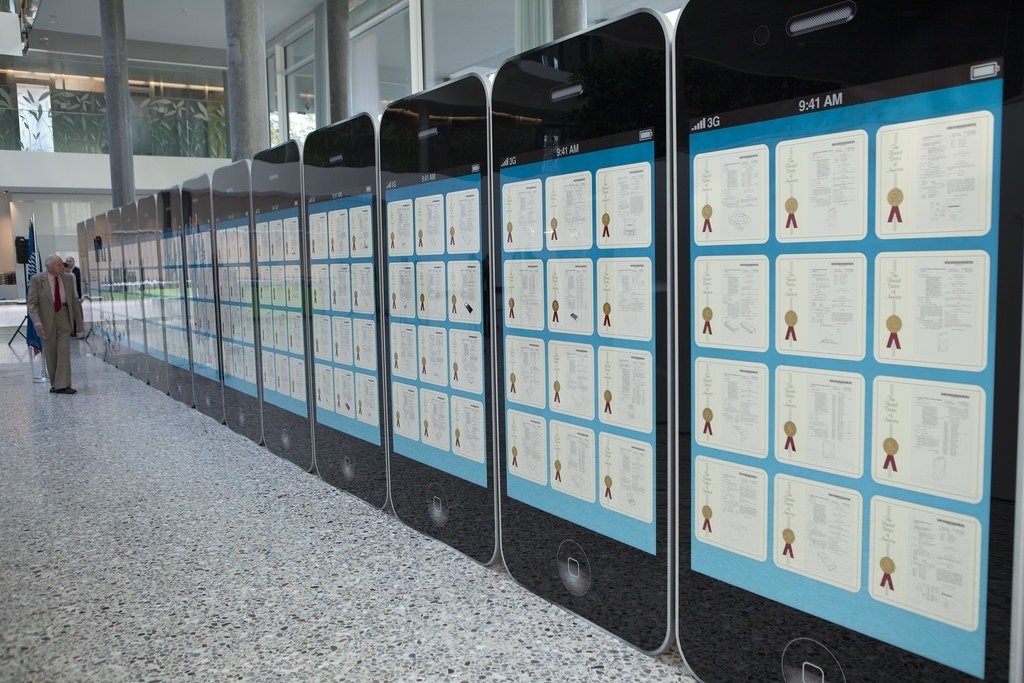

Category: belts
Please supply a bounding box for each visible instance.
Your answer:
[63,303,67,306]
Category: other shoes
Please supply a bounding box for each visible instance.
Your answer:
[50,388,55,392]
[55,387,77,394]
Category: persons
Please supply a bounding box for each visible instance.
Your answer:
[66,256,82,299]
[27,255,84,394]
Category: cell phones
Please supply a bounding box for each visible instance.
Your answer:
[77,1,1024,683]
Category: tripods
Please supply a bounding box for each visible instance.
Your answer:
[8,264,30,347]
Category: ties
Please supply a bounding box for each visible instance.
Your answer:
[54,276,62,311]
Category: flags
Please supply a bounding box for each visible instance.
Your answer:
[25,221,43,356]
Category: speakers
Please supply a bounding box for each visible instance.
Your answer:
[14,237,30,264]
